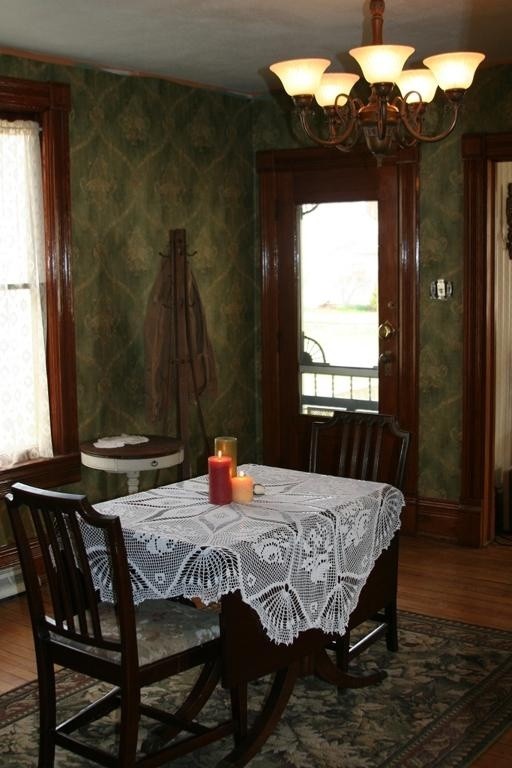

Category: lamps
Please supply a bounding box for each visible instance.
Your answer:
[268,2,486,165]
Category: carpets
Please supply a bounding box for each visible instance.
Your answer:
[3,610,510,766]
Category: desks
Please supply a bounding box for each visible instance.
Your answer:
[78,463,396,764]
[80,434,198,607]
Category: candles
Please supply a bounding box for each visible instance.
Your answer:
[208,452,232,505]
[233,471,253,505]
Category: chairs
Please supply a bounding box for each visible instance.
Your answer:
[2,483,247,765]
[273,411,405,693]
